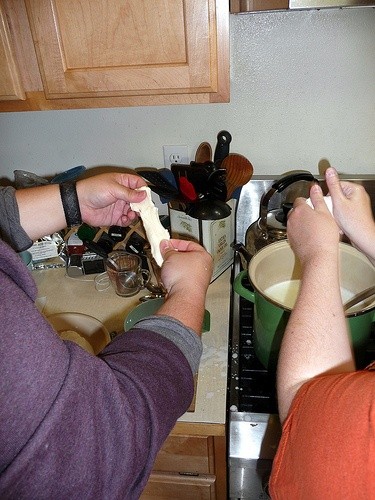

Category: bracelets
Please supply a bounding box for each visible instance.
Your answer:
[58,181,83,228]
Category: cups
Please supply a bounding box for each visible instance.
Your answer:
[104,252,151,297]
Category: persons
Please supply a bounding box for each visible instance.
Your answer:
[0,171,214,500]
[267,167,375,500]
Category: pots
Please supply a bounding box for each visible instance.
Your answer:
[234,239,374,373]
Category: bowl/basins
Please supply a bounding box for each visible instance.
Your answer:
[46,312,114,355]
[18,251,33,272]
[124,297,165,333]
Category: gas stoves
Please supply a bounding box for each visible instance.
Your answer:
[229,175,374,456]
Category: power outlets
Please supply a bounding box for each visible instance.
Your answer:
[163,144,190,172]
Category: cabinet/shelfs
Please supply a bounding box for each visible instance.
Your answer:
[0,0,231,113]
[22,419,228,500]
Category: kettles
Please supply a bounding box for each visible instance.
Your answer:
[230,170,352,269]
